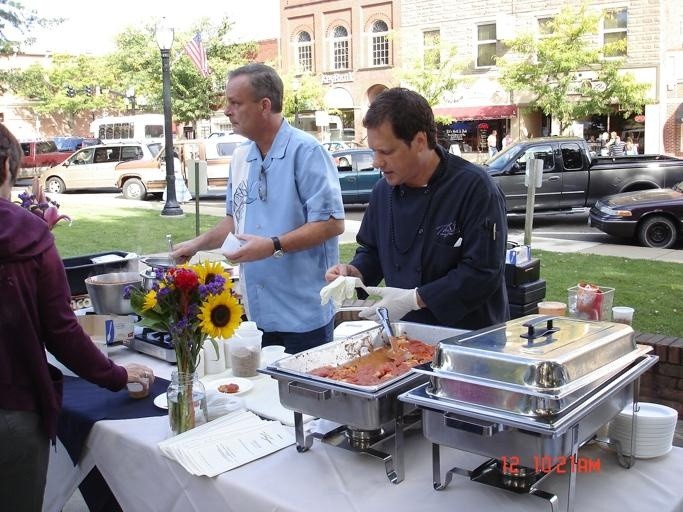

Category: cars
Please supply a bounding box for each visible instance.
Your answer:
[109,128,385,210]
[584,178,682,251]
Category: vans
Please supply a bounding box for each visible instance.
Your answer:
[15,132,109,182]
[40,138,164,201]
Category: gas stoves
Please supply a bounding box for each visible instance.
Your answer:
[129,328,178,364]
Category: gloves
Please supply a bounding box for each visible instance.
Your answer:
[356,284,419,326]
[318,273,364,309]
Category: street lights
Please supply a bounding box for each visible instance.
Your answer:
[152,15,187,216]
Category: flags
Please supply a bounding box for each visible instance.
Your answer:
[183,33,209,78]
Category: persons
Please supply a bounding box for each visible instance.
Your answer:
[487,129,498,158]
[156,137,192,204]
[501,134,512,150]
[169,64,345,355]
[322,86,510,371]
[588,130,639,157]
[1,122,156,512]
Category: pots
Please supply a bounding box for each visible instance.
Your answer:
[141,255,180,295]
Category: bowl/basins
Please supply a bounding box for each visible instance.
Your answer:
[84,271,143,315]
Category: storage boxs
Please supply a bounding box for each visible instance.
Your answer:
[58,249,146,297]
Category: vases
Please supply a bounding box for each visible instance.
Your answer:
[164,368,210,435]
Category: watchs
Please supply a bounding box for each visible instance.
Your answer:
[271,237,285,258]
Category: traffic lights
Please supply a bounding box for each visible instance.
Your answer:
[84,84,93,96]
[65,84,75,98]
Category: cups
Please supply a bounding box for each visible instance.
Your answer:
[576,280,599,314]
[612,307,636,328]
[200,320,264,378]
[537,301,566,319]
[127,377,149,400]
[263,345,286,362]
[220,231,241,255]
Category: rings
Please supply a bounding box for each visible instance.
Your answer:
[145,371,148,378]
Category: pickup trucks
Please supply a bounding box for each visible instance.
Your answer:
[470,132,682,218]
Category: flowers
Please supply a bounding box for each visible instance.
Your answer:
[9,176,71,233]
[119,251,243,433]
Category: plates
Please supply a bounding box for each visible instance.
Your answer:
[209,377,251,395]
[607,400,680,460]
[153,392,168,410]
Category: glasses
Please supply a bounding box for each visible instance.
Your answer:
[256,163,268,203]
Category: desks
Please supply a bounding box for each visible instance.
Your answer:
[39,300,683,512]
